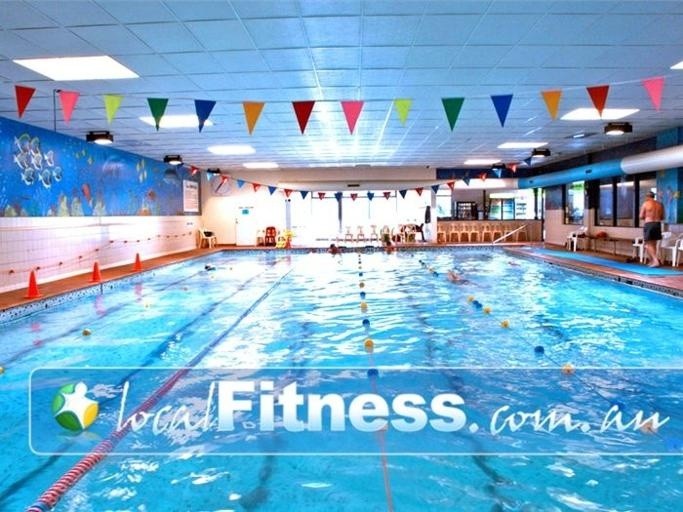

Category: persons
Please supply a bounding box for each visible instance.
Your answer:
[328,244,335,252]
[639,191,665,267]
[386,241,395,255]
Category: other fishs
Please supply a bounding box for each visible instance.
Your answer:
[11,131,183,215]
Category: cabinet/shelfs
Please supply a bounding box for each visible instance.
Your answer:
[454,196,527,221]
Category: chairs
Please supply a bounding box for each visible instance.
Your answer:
[255,226,295,249]
[566,224,587,252]
[341,222,530,245]
[197,227,216,250]
[630,230,682,269]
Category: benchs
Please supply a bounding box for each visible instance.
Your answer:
[585,234,641,262]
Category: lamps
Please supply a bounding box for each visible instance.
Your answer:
[602,121,634,135]
[531,148,550,159]
[162,154,182,167]
[84,129,115,148]
[207,168,220,176]
